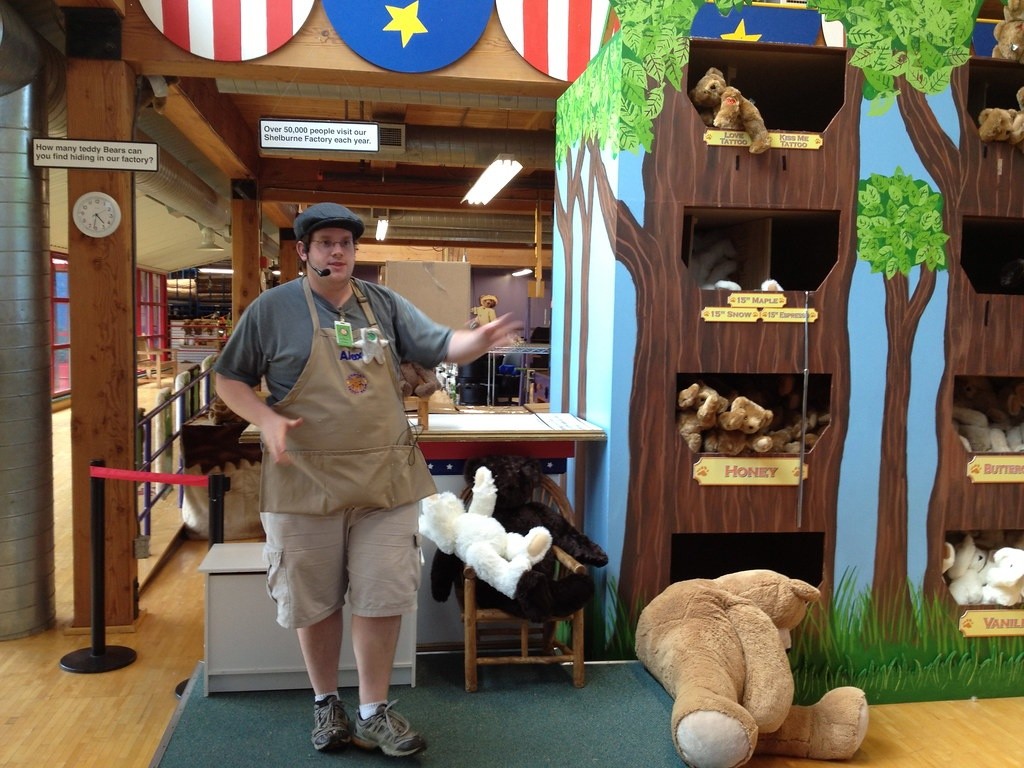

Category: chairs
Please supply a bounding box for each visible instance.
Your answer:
[136,337,179,388]
[459,468,585,690]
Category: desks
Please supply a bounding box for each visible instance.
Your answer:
[238,413,606,655]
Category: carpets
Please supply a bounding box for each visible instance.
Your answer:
[149,658,687,768]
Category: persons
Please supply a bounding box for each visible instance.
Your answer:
[212,201,520,757]
[470,294,498,330]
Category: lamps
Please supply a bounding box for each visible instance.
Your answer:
[375,215,389,242]
[460,152,523,205]
[196,227,224,252]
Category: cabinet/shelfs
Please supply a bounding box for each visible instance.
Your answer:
[197,544,418,697]
[551,0,1024,710]
[481,343,551,406]
[169,324,231,354]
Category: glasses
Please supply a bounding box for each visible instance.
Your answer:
[310,237,355,251]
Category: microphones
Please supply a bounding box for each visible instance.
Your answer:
[307,252,330,277]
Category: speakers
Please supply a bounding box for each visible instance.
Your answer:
[230,178,257,199]
[62,7,121,60]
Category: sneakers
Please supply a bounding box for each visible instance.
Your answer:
[312,696,353,750]
[352,699,427,756]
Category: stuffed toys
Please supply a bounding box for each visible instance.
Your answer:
[397,358,442,398]
[976,0,1024,146]
[635,567,869,768]
[943,530,1024,607]
[691,237,783,292]
[689,68,771,154]
[416,452,607,620]
[951,376,1024,452]
[674,381,831,454]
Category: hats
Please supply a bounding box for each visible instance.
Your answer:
[293,202,364,242]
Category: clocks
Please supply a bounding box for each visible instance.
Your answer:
[73,191,122,239]
